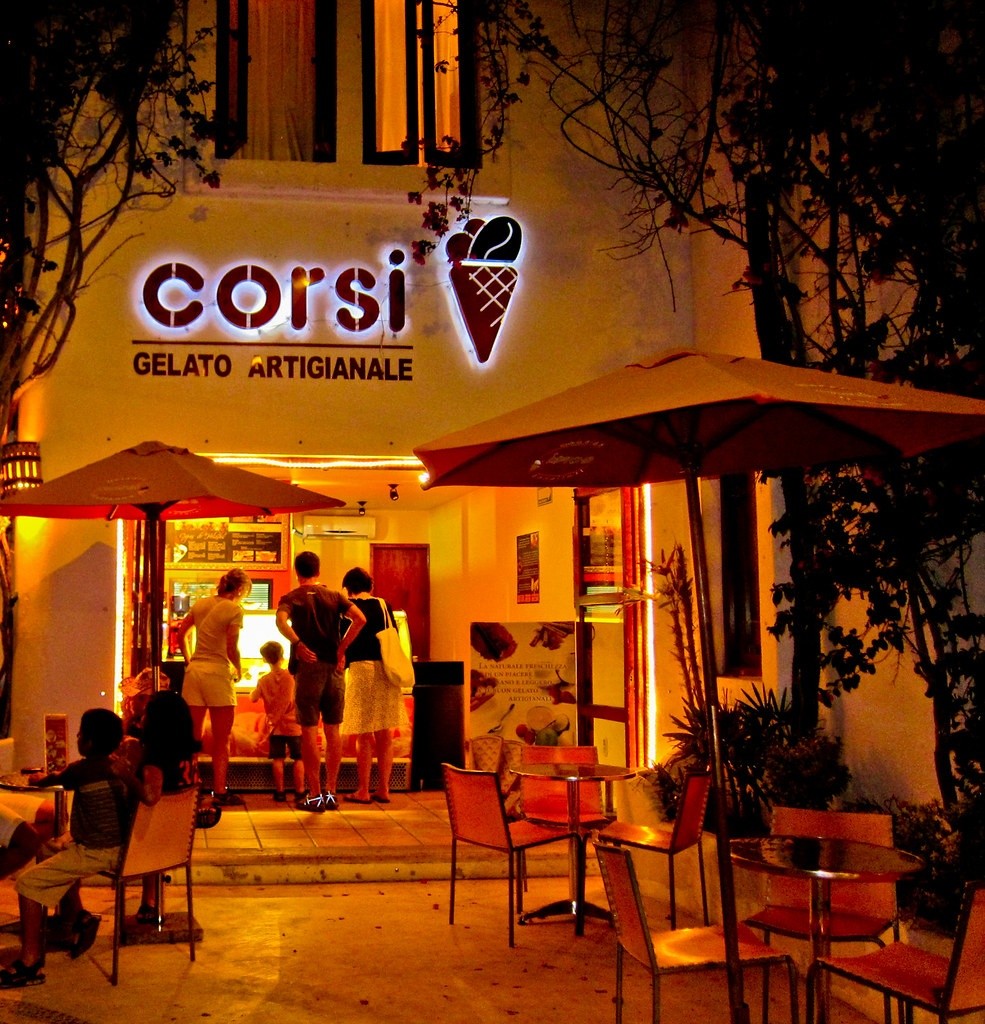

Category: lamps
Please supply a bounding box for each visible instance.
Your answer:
[356,500,367,515]
[388,483,399,499]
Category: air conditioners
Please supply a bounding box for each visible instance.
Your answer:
[301,515,376,540]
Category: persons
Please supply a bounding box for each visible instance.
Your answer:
[178,568,253,804]
[249,552,409,813]
[0,667,222,988]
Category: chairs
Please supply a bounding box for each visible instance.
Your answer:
[589,842,799,1024]
[518,742,610,892]
[78,783,202,986]
[440,761,583,945]
[805,879,985,1024]
[599,771,713,931]
[741,807,906,1023]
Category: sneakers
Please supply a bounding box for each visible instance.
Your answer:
[295,792,327,811]
[321,791,339,810]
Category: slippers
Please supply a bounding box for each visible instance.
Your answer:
[369,792,391,803]
[346,792,372,804]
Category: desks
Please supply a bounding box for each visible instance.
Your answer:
[508,759,638,937]
[0,771,78,953]
[733,836,926,1024]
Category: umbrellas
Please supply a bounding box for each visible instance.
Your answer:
[412,349,985,1024]
[1,442,347,724]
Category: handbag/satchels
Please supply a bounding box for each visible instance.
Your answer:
[376,595,415,688]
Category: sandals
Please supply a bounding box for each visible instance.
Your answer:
[0,956,46,988]
[66,909,99,961]
[213,790,246,806]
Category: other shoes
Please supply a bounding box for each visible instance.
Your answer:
[136,899,165,924]
[272,791,287,802]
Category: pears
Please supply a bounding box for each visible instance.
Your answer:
[535,727,558,746]
